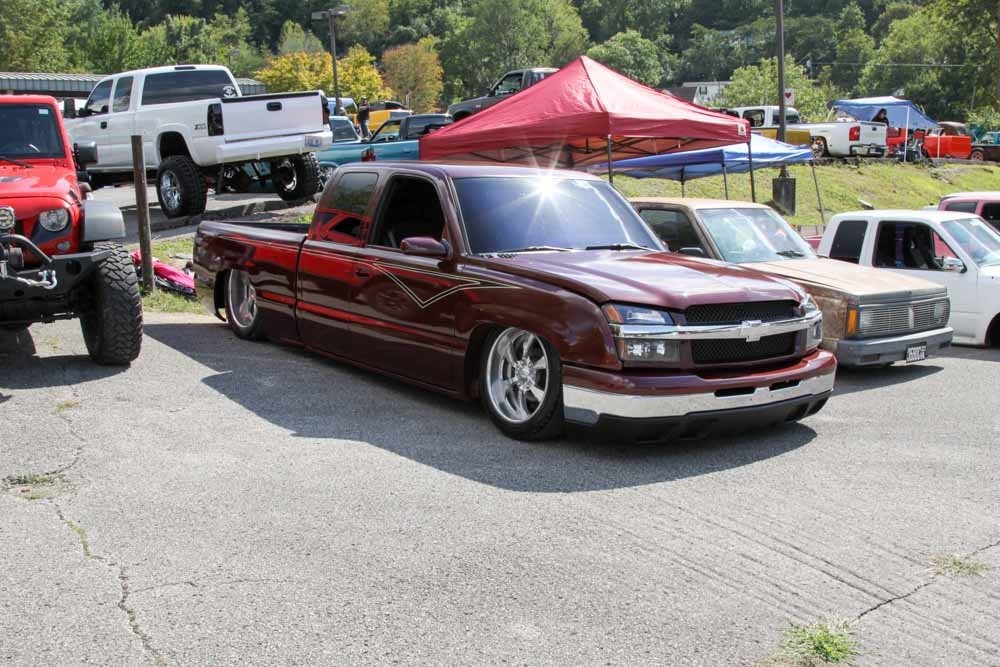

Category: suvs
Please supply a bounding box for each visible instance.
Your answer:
[0,95,144,365]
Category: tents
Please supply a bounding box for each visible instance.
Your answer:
[419,55,751,181]
[592,131,828,233]
[833,95,940,129]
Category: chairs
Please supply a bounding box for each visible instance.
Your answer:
[384,187,433,250]
[903,226,944,270]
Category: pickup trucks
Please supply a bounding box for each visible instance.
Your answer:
[804,191,1000,348]
[191,162,837,442]
[63,64,334,219]
[728,106,1000,161]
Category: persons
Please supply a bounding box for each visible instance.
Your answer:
[335,98,346,116]
[356,96,370,137]
[870,108,890,129]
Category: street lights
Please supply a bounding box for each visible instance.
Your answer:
[311,6,348,116]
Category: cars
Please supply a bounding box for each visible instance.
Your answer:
[243,67,561,192]
[628,197,954,368]
